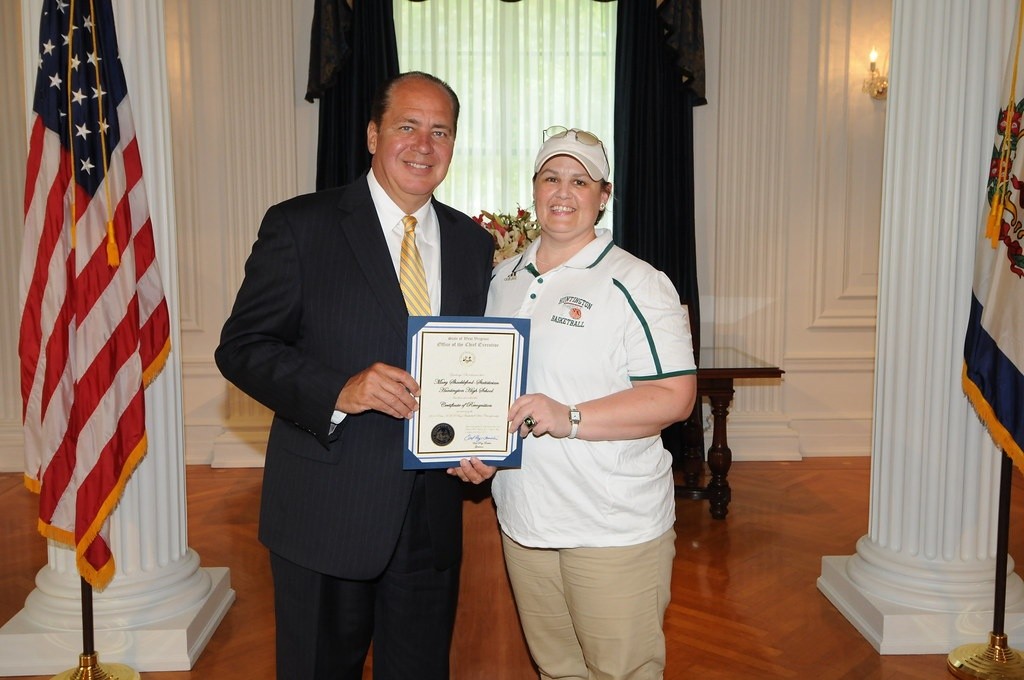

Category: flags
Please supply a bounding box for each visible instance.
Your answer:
[960,0,1024,480]
[18,0,173,592]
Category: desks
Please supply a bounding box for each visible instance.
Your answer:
[674,347,786,520]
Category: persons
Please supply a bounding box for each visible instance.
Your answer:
[214,69,497,680]
[482,124,698,680]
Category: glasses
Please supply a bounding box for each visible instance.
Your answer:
[543,126,606,150]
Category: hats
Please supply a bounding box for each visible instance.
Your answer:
[534,129,611,181]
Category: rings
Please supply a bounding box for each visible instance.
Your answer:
[523,416,536,428]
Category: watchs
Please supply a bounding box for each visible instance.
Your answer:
[568,405,581,439]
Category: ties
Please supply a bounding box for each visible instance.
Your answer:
[399,217,431,316]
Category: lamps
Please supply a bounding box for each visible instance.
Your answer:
[861,47,887,101]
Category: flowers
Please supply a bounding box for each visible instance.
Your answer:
[472,202,541,269]
